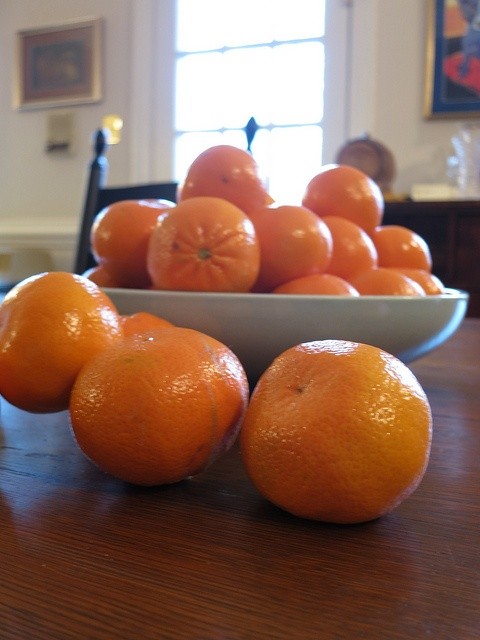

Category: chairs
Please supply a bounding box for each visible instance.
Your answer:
[71,115,260,276]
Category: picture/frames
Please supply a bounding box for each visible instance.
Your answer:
[420,2,478,119]
[13,17,103,112]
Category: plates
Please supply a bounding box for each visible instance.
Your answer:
[99,285,470,387]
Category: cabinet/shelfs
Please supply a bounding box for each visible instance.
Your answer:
[372,200,479,317]
